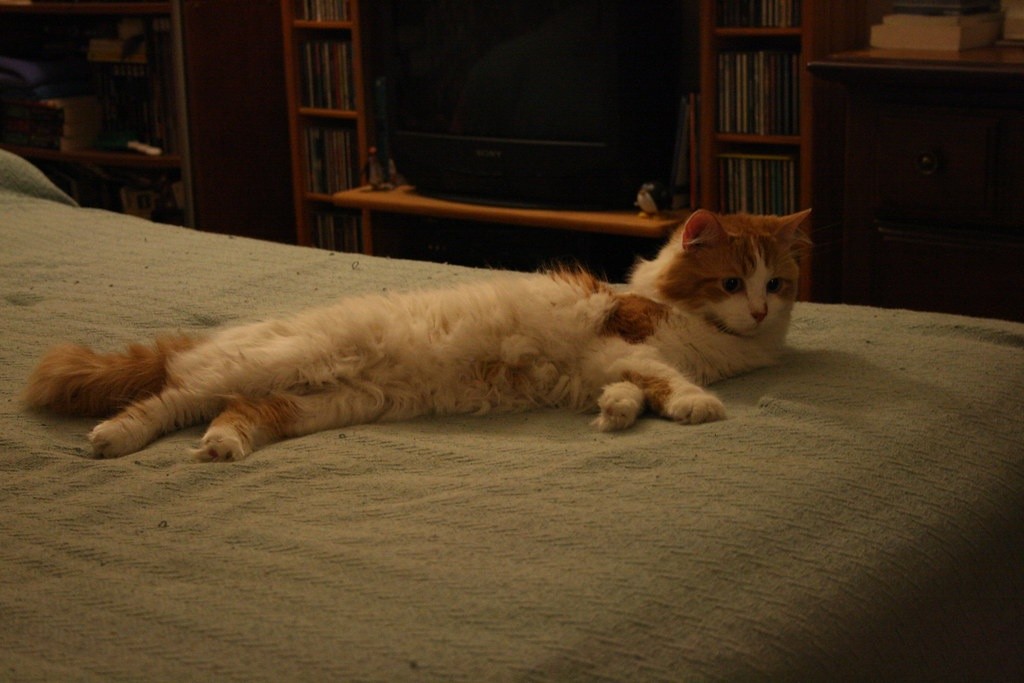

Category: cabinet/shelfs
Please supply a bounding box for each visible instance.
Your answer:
[0,0,297,242]
[808,47,1024,320]
[694,0,853,301]
[280,0,380,255]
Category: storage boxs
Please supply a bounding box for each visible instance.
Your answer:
[871,21,1002,54]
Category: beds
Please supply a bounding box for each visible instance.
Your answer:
[0,148,1024,683]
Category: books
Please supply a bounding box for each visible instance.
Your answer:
[713,0,802,29]
[301,0,349,22]
[314,210,362,253]
[869,0,1001,54]
[302,125,353,195]
[716,152,796,216]
[5,95,104,151]
[302,39,351,112]
[718,49,801,136]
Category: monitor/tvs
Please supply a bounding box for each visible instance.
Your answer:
[360,0,688,210]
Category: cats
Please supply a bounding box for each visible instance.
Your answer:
[26,203,814,461]
